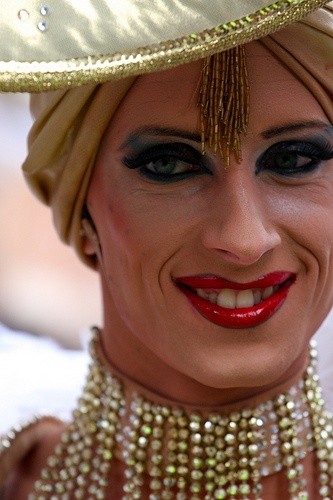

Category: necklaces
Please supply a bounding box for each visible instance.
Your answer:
[24,327,332,500]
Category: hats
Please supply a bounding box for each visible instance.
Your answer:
[0,0,333,270]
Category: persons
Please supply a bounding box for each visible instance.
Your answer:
[0,0,333,500]
